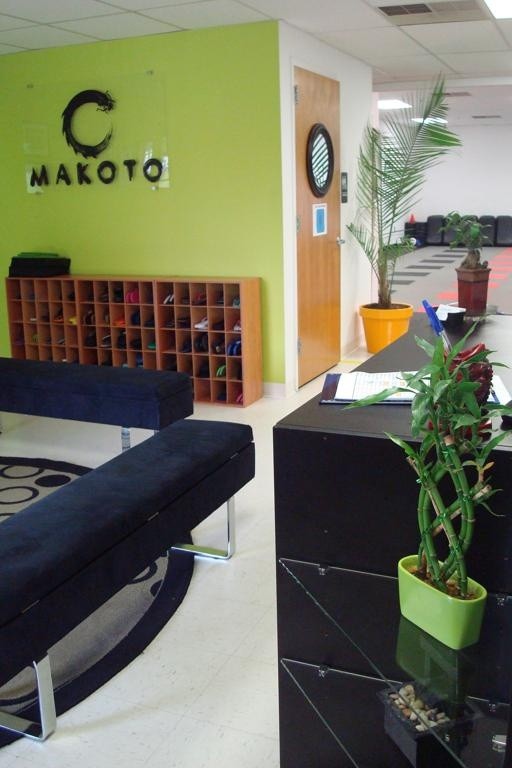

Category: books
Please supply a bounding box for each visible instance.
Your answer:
[319,372,512,407]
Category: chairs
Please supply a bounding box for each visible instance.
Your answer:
[403,213,511,248]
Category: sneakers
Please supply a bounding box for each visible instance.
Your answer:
[15,288,245,404]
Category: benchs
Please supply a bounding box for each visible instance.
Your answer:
[0,420,257,745]
[0,356,193,455]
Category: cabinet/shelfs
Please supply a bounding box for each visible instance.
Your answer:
[5,274,266,407]
[271,310,512,768]
[278,551,512,765]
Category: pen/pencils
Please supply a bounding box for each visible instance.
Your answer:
[421,299,455,358]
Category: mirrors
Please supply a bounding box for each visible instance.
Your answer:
[305,122,335,196]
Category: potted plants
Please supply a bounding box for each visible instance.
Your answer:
[345,309,510,645]
[433,208,492,318]
[343,68,463,354]
[393,617,475,751]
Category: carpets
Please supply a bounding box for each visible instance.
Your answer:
[0,459,197,750]
